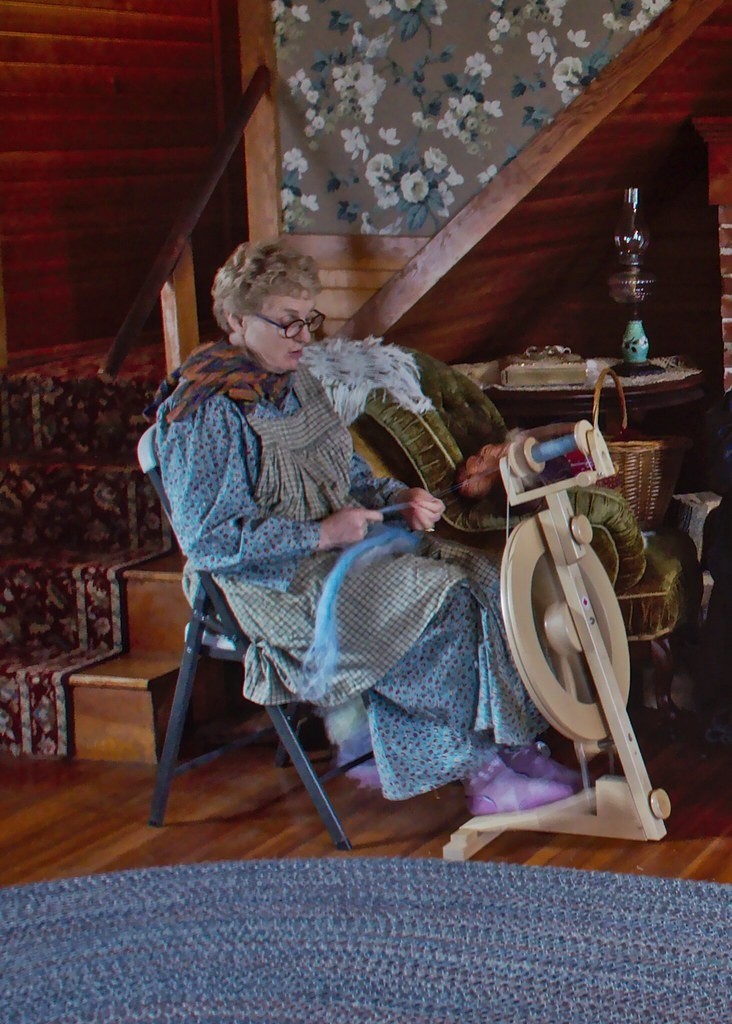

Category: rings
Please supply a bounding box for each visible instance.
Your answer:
[425,528,435,531]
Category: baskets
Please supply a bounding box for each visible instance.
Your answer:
[593,366,681,531]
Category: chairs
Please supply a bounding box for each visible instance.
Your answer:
[137,424,462,851]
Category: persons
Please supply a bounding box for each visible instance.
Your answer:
[150,239,587,815]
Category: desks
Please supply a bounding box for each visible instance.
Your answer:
[449,356,708,679]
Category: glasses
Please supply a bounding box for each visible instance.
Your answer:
[253,308,326,339]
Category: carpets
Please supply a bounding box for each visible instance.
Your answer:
[2,855,731,1022]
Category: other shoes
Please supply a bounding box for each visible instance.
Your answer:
[461,753,574,816]
[498,742,595,793]
